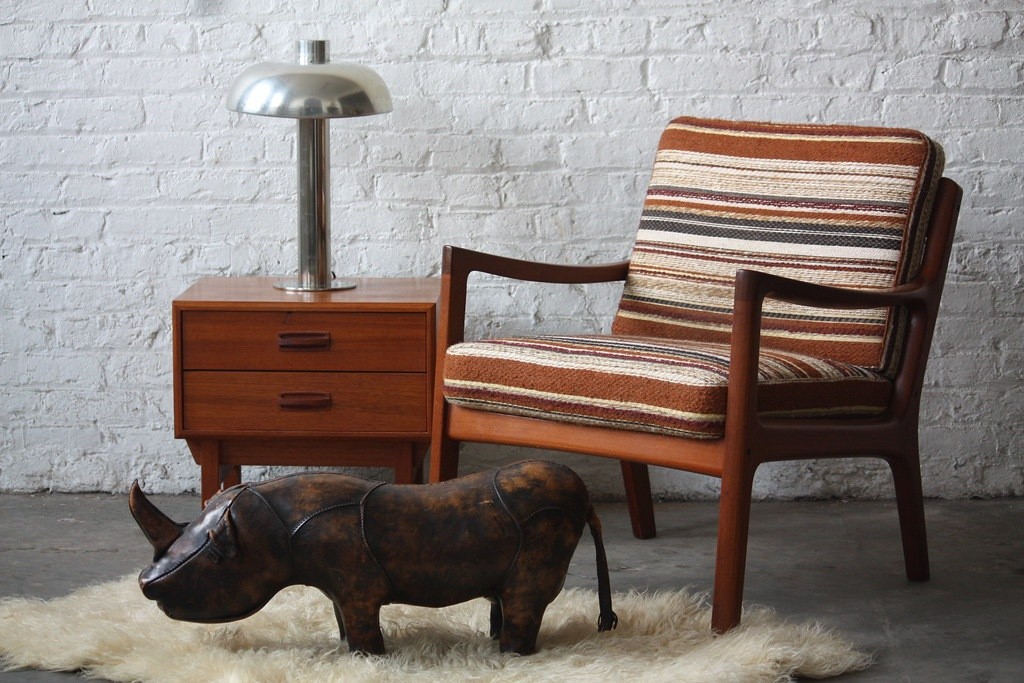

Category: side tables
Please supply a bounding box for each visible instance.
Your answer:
[173,277,441,513]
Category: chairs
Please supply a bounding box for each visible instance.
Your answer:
[429,116,963,640]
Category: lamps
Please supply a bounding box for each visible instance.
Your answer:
[226,39,394,291]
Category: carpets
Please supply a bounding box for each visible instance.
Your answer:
[0,566,878,683]
